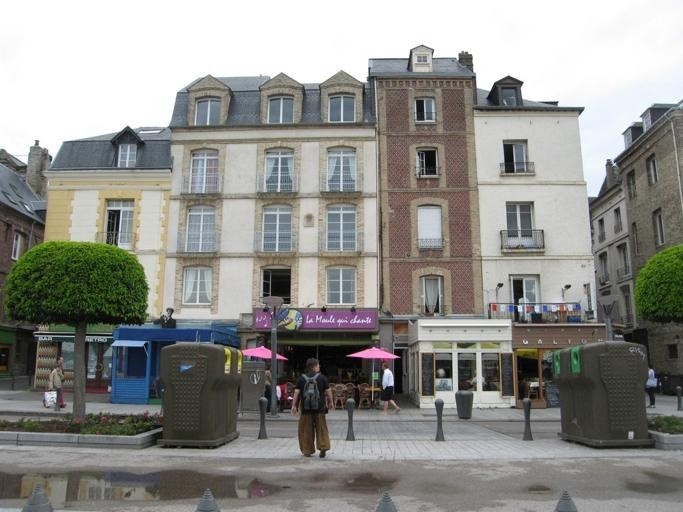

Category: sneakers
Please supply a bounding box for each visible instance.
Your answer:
[319,448,326,457]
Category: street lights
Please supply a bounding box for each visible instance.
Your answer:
[259,295,285,416]
[596,293,621,341]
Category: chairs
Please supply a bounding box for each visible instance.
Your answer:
[521,376,546,400]
[277,381,381,411]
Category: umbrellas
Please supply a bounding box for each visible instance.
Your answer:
[241,346,289,361]
[346,347,401,401]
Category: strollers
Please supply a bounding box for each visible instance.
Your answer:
[275,382,296,413]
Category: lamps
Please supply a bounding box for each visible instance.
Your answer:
[561,283,571,298]
[262,302,269,314]
[495,282,502,296]
[349,306,355,312]
[321,306,326,312]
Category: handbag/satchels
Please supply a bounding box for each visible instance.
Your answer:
[380,391,393,401]
[44,390,57,408]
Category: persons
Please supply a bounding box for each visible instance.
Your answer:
[645,363,657,408]
[290,358,335,458]
[264,370,273,412]
[154,308,176,350]
[43,356,67,408]
[380,363,400,416]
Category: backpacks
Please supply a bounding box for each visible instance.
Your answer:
[301,374,321,412]
